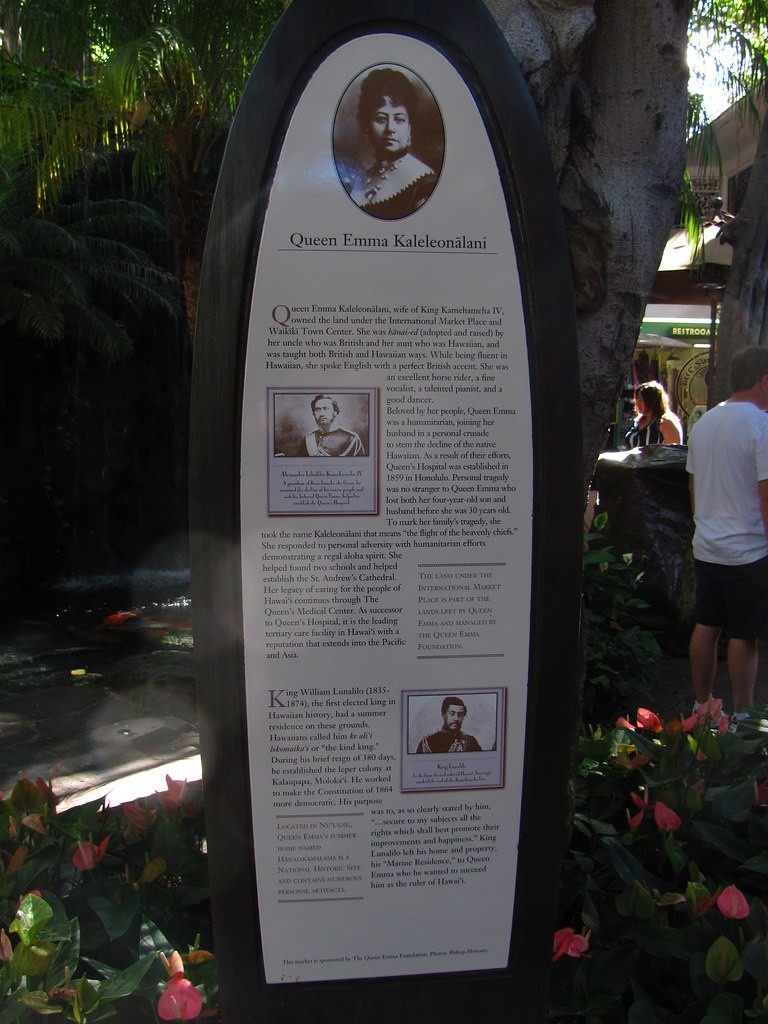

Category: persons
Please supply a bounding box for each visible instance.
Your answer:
[685,344,768,734]
[625,380,683,450]
[350,68,438,219]
[295,395,366,457]
[416,697,483,753]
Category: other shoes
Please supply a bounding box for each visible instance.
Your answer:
[693,708,728,730]
[730,714,752,735]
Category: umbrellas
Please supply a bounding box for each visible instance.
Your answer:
[626,333,693,397]
[657,197,735,411]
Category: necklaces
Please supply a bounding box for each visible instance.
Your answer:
[364,149,408,200]
